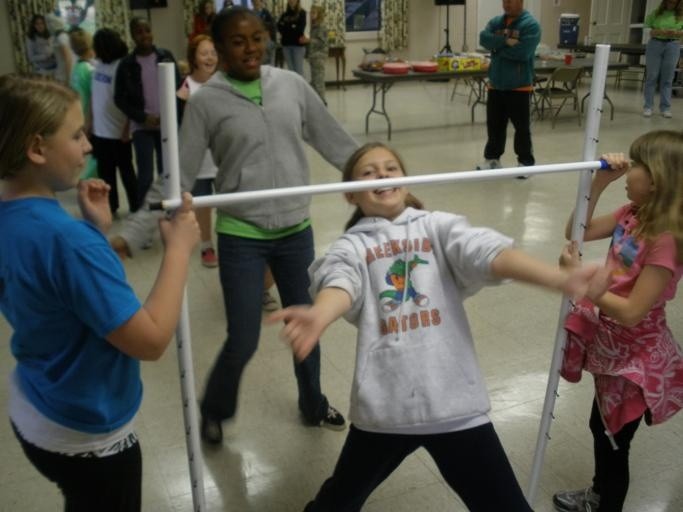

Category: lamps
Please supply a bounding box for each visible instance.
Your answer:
[435,0,466,54]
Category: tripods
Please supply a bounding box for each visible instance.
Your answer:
[440,5,452,54]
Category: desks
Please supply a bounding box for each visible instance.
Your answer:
[352,56,629,142]
[578,42,683,92]
[275,46,347,92]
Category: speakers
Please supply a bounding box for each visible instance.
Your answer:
[435,0,465,5]
[130,0,168,10]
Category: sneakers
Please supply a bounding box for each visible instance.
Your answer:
[477,160,502,169]
[644,109,672,118]
[322,406,344,429]
[553,487,600,512]
[262,293,278,311]
[201,247,218,266]
[201,415,222,445]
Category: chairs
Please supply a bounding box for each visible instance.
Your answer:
[449,49,647,129]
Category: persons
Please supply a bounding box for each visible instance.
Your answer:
[261,141,616,512]
[24,1,329,312]
[476,0,541,179]
[110,11,360,446]
[551,130,683,511]
[0,71,201,511]
[642,0,682,118]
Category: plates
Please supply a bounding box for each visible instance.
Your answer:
[383,62,439,73]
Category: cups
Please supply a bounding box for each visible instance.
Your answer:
[564,53,572,64]
